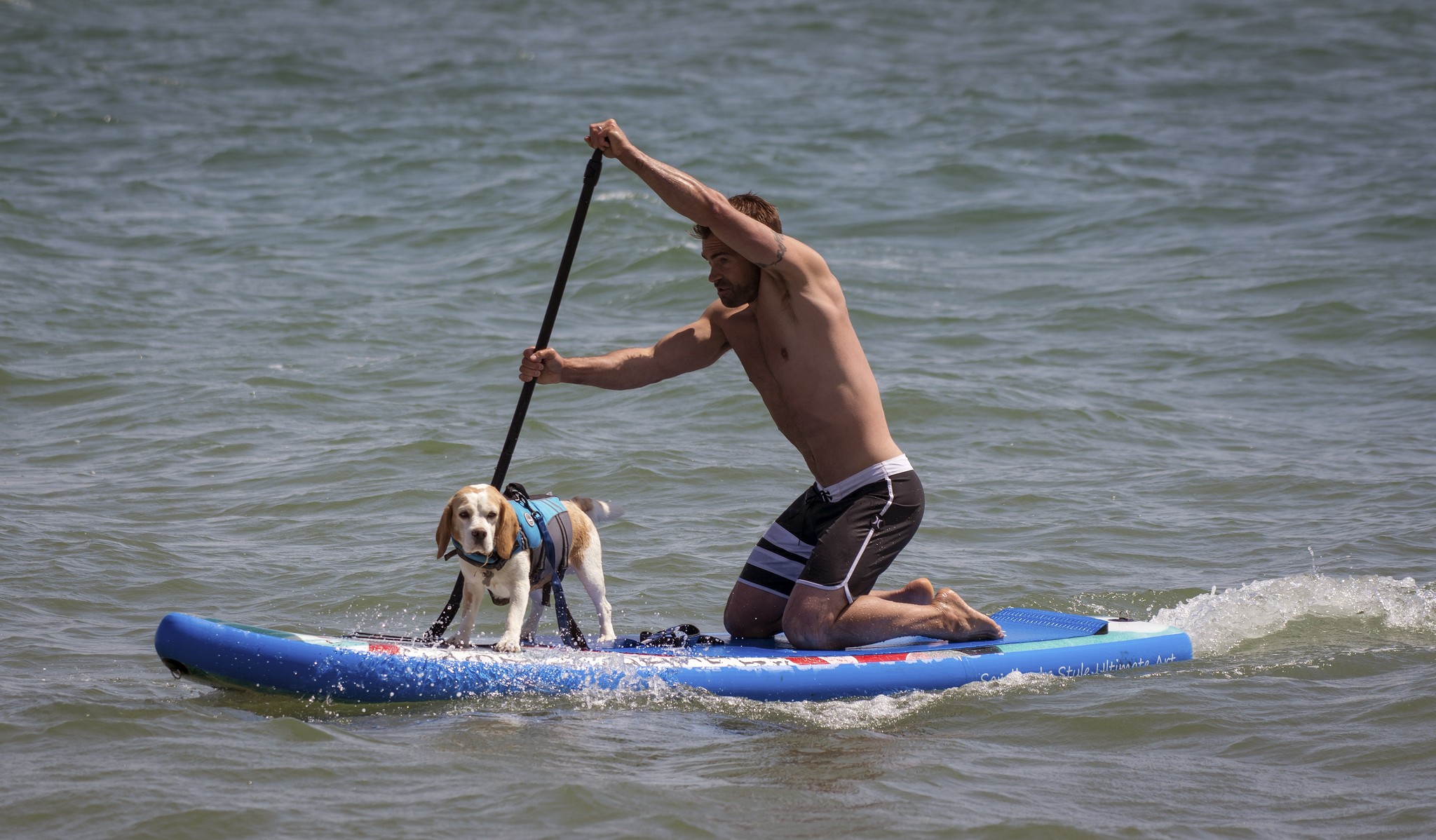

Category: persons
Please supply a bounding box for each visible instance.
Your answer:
[518,116,1005,651]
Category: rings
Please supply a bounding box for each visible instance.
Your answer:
[597,126,603,130]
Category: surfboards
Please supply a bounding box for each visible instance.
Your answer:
[152,602,1197,697]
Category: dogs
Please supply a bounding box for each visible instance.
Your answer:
[433,482,627,654]
